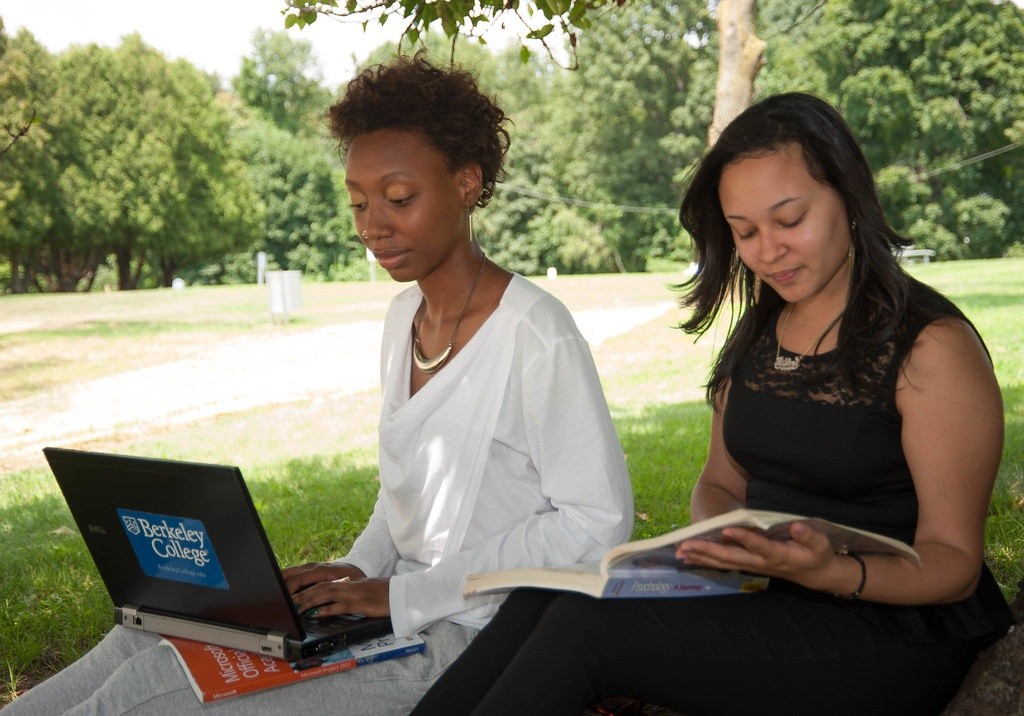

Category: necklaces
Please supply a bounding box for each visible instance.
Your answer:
[403,249,487,375]
[773,300,846,371]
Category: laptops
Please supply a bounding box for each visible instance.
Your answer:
[39,447,394,663]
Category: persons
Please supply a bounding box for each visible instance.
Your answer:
[0,54,637,716]
[398,93,1006,716]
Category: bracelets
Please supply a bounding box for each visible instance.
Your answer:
[844,553,867,599]
